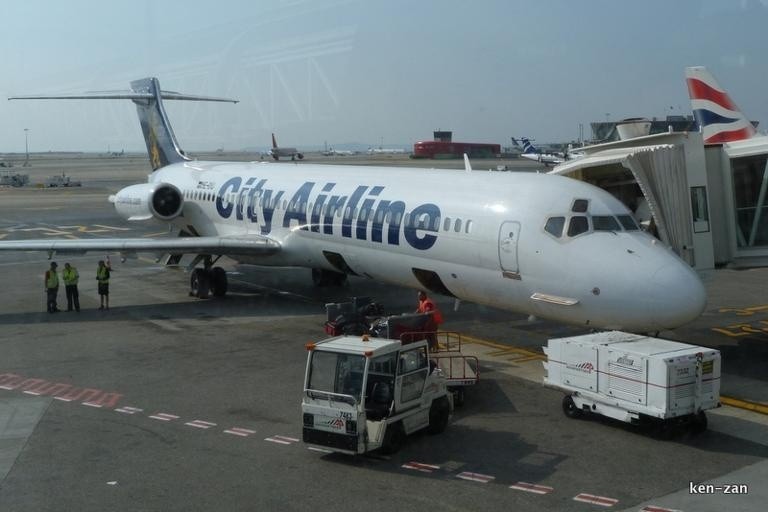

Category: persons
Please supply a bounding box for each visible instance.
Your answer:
[43,262,59,312]
[63,262,81,313]
[416,289,445,327]
[95,260,109,311]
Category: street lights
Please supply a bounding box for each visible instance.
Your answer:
[23,128,30,158]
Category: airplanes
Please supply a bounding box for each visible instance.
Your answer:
[266,131,303,162]
[511,134,589,167]
[321,141,405,156]
[0,75,709,338]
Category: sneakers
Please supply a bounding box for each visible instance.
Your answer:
[46,307,109,312]
